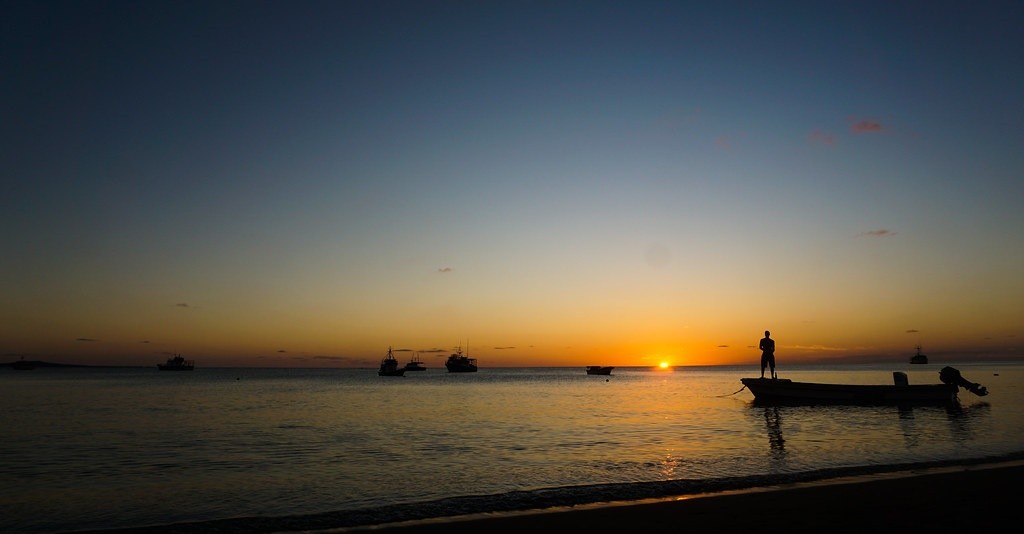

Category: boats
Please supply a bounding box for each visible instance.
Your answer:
[741,366,989,406]
[378,345,407,376]
[444,339,478,373]
[404,352,426,371]
[909,344,928,364]
[584,366,615,375]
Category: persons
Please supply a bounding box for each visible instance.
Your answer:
[759,331,775,380]
